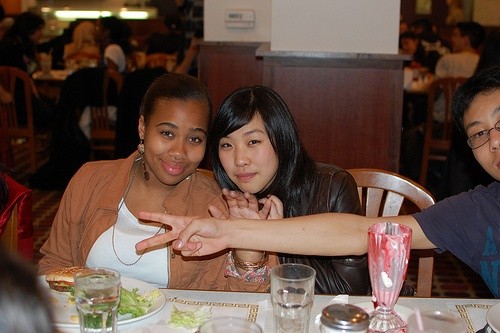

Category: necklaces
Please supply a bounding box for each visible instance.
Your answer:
[111,162,164,265]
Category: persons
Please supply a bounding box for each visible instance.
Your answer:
[36,74,282,295]
[398,0,500,172]
[208,86,372,297]
[0,242,60,333]
[136,68,500,300]
[0,0,205,179]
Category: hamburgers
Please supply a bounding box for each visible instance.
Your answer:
[45,265,90,295]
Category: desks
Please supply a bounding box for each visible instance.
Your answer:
[30,66,68,99]
[53,286,500,333]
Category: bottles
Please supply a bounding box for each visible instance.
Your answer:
[319,303,369,333]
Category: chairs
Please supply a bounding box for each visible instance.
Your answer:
[420,75,470,188]
[0,64,59,180]
[345,168,439,298]
[88,65,126,160]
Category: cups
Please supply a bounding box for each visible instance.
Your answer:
[194,315,263,333]
[270,263,315,333]
[73,267,122,333]
[365,222,412,331]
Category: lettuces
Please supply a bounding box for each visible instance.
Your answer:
[69,284,150,328]
[170,305,211,329]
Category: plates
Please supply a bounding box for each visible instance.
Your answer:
[315,303,413,333]
[40,275,166,329]
[486,301,500,333]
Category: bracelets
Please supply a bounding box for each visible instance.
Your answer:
[232,248,267,271]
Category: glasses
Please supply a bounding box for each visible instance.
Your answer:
[466,119,500,150]
